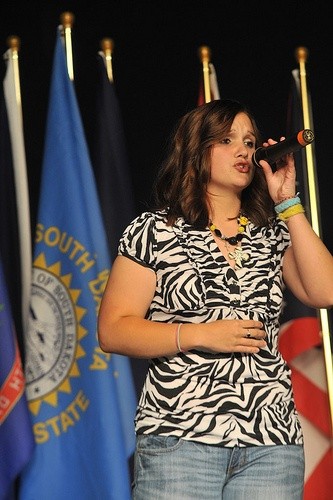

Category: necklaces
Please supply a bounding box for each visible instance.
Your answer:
[206,209,250,245]
[211,229,250,270]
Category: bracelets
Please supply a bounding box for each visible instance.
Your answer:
[275,197,301,212]
[274,194,297,206]
[176,322,184,353]
[278,204,305,220]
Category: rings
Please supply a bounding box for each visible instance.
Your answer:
[247,329,251,337]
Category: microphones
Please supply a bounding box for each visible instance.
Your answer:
[252,129,315,169]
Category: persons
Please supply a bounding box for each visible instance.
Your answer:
[98,97,333,500]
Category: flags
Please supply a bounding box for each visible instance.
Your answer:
[0,50,38,500]
[281,68,333,500]
[93,55,141,262]
[18,32,140,500]
